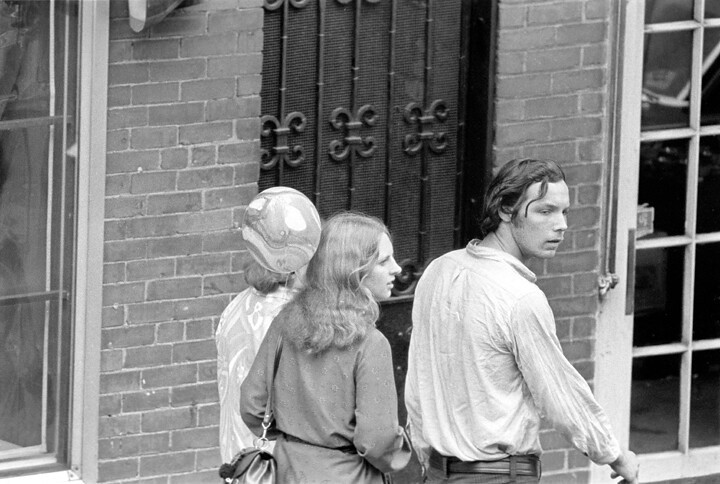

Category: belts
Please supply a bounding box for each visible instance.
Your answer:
[428,449,536,476]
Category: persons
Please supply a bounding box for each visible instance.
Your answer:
[239,213,412,484]
[404,157,641,484]
[216,186,322,484]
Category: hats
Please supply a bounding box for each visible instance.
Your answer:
[242,186,321,273]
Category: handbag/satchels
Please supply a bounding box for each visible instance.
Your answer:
[224,447,278,484]
